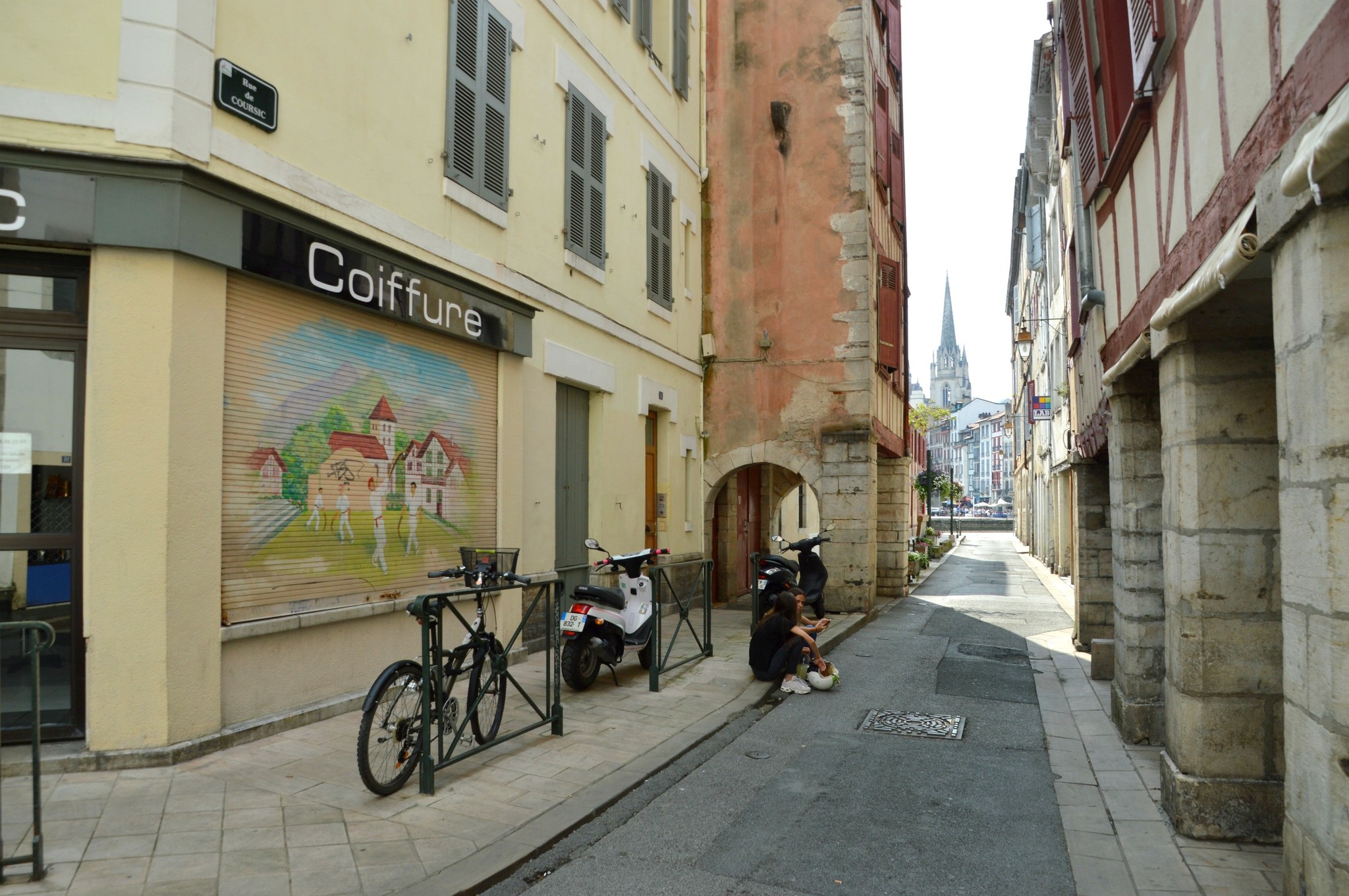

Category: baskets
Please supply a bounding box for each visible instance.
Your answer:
[458,544,520,588]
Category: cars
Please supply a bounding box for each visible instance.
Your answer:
[925,507,946,517]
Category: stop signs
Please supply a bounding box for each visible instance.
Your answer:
[966,497,971,502]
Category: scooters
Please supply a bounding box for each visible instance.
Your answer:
[555,537,671,692]
[753,523,837,626]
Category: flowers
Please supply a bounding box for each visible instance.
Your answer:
[915,538,934,554]
[924,527,943,538]
[908,551,930,582]
[909,464,964,508]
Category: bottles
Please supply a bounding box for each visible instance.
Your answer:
[796,651,810,681]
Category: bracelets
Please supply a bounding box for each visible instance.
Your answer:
[815,656,821,660]
[815,626,818,633]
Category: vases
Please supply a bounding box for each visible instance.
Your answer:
[926,535,937,546]
[917,546,928,555]
[908,558,921,576]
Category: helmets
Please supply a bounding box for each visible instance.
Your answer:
[806,659,839,690]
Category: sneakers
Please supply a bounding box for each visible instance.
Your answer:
[781,676,811,694]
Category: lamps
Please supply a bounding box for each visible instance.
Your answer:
[1004,415,1027,439]
[998,445,1021,461]
[1015,317,1067,364]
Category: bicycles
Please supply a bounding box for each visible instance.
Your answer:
[357,545,532,795]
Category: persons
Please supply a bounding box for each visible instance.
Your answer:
[749,592,827,694]
[939,505,1011,518]
[762,587,831,660]
